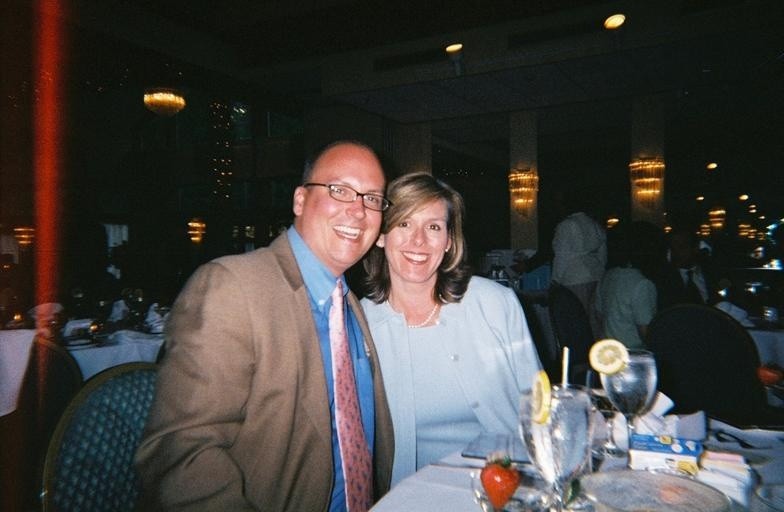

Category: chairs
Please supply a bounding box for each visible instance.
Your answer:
[38,358,159,512]
[548,281,594,378]
[641,302,764,427]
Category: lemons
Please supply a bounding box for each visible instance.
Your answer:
[531,368,552,423]
[589,339,629,375]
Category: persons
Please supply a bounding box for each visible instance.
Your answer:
[512,184,724,386]
[355,170,547,493]
[130,137,397,512]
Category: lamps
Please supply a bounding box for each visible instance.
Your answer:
[142,85,187,118]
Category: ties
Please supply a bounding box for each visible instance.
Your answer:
[329,280,373,511]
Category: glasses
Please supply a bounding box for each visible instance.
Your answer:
[304,183,393,212]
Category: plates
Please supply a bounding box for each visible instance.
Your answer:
[51,338,99,353]
[23,303,65,321]
[581,467,736,512]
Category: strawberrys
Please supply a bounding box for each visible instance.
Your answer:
[480,458,521,511]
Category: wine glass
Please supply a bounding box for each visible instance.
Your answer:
[598,346,658,469]
[66,289,167,339]
[516,388,593,510]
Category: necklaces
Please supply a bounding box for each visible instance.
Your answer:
[387,288,443,328]
